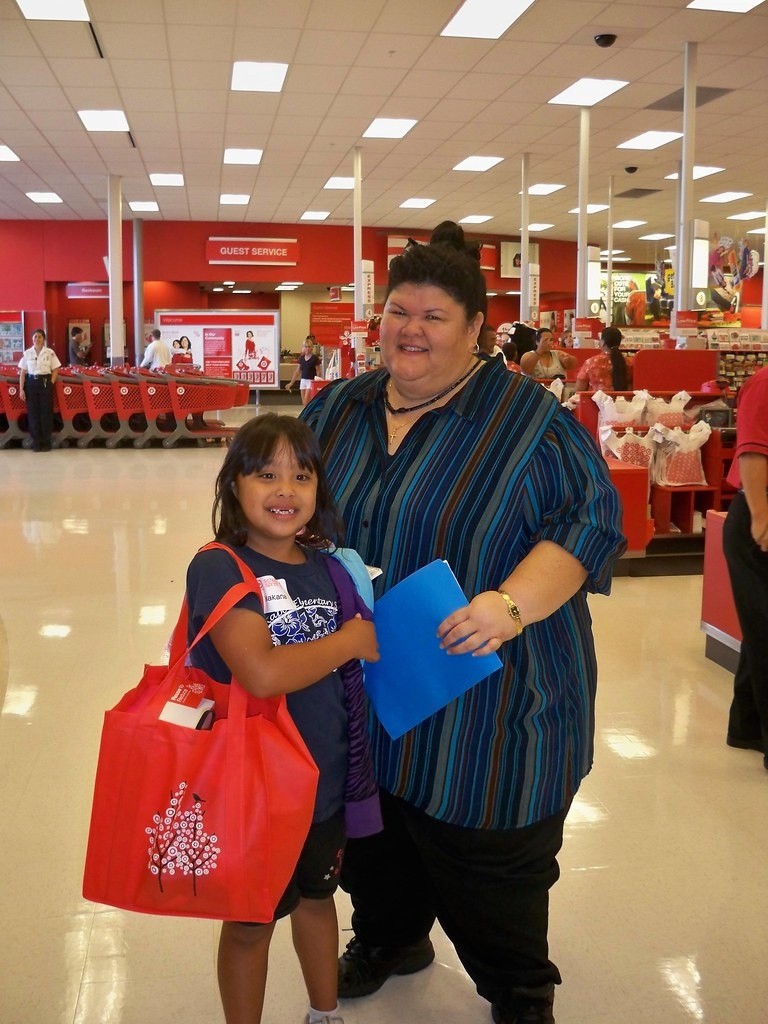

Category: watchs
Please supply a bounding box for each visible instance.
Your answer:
[498,590,523,638]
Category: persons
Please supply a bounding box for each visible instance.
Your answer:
[171,336,193,365]
[182,412,381,1024]
[18,329,62,453]
[244,331,256,361]
[295,219,628,1024]
[139,329,169,374]
[68,327,94,367]
[285,339,322,410]
[720,364,768,772]
[476,323,634,396]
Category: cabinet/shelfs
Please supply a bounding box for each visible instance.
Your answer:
[517,317,768,552]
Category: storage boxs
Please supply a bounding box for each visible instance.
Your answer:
[351,320,382,375]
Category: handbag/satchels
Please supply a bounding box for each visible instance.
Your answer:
[82,542,321,924]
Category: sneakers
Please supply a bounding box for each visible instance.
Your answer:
[337,928,435,999]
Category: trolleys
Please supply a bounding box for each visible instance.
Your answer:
[0,363,252,449]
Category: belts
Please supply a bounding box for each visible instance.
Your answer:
[27,374,52,379]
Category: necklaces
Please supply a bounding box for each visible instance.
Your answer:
[378,357,483,414]
[385,380,441,447]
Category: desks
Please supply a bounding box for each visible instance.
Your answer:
[280,363,300,380]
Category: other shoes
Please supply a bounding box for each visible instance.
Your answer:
[305,1014,344,1024]
